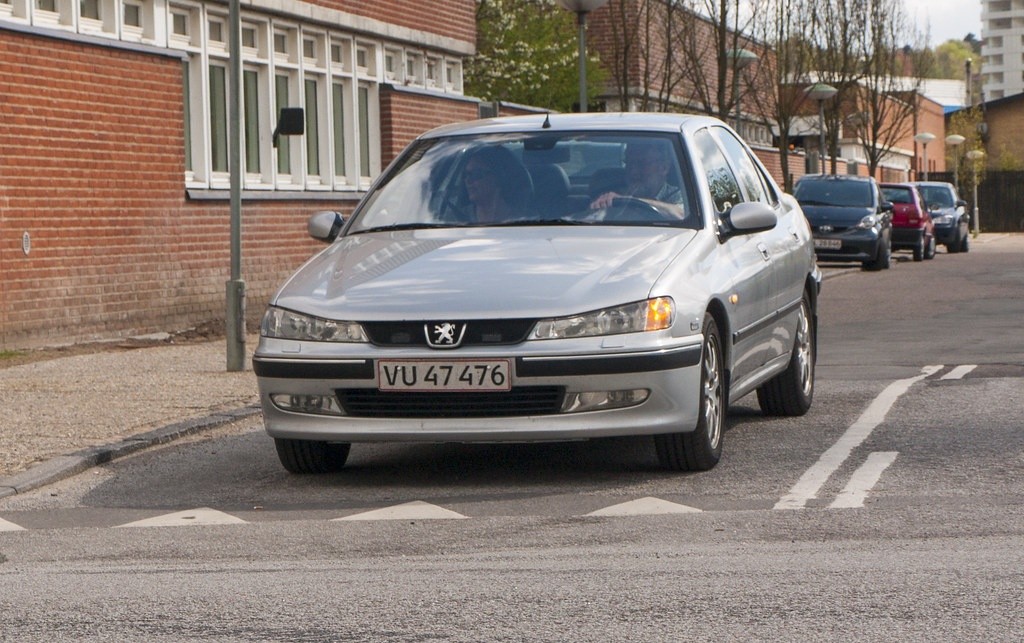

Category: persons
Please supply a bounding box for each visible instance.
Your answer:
[588,141,685,223]
[442,144,539,226]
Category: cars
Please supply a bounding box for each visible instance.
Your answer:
[917,180,972,255]
[878,181,937,262]
[791,173,892,274]
[251,112,825,472]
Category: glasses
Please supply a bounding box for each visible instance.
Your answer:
[462,168,491,180]
[622,158,658,170]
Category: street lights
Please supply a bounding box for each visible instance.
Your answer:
[726,46,758,136]
[561,1,608,112]
[803,82,839,173]
[967,148,987,238]
[946,132,965,191]
[847,110,872,173]
[912,130,937,179]
